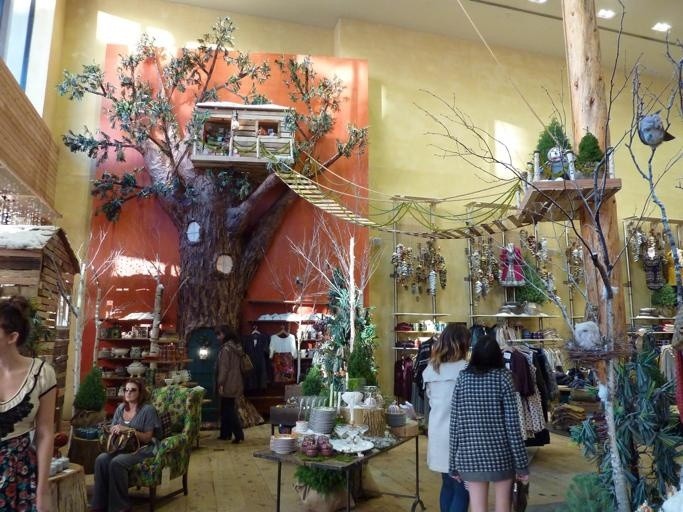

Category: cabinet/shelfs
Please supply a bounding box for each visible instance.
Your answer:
[94,316,159,408]
[247,298,329,384]
[394,313,450,411]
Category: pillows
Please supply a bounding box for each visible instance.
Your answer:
[157,412,171,439]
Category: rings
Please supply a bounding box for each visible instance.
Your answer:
[118,431,120,433]
[115,431,117,433]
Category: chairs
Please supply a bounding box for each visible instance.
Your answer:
[99,386,205,512]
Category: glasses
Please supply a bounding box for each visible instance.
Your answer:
[125,387,136,392]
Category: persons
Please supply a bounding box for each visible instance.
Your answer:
[449,335,529,512]
[638,236,666,292]
[420,323,471,512]
[91,378,162,512]
[500,242,527,289]
[214,325,245,443]
[0,295,58,512]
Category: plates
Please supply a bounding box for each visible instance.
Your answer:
[309,407,336,435]
[270,434,297,455]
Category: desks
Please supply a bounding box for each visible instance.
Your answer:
[47,462,85,512]
[251,429,426,512]
[269,406,300,435]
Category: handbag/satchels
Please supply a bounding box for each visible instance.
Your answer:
[512,481,527,512]
[107,428,140,453]
[241,354,254,370]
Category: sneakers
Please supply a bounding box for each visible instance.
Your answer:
[217,436,231,440]
[232,436,244,443]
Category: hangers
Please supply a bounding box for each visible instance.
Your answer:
[275,325,289,337]
[250,325,261,335]
[500,340,531,356]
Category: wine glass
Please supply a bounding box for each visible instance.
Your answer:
[334,424,369,450]
